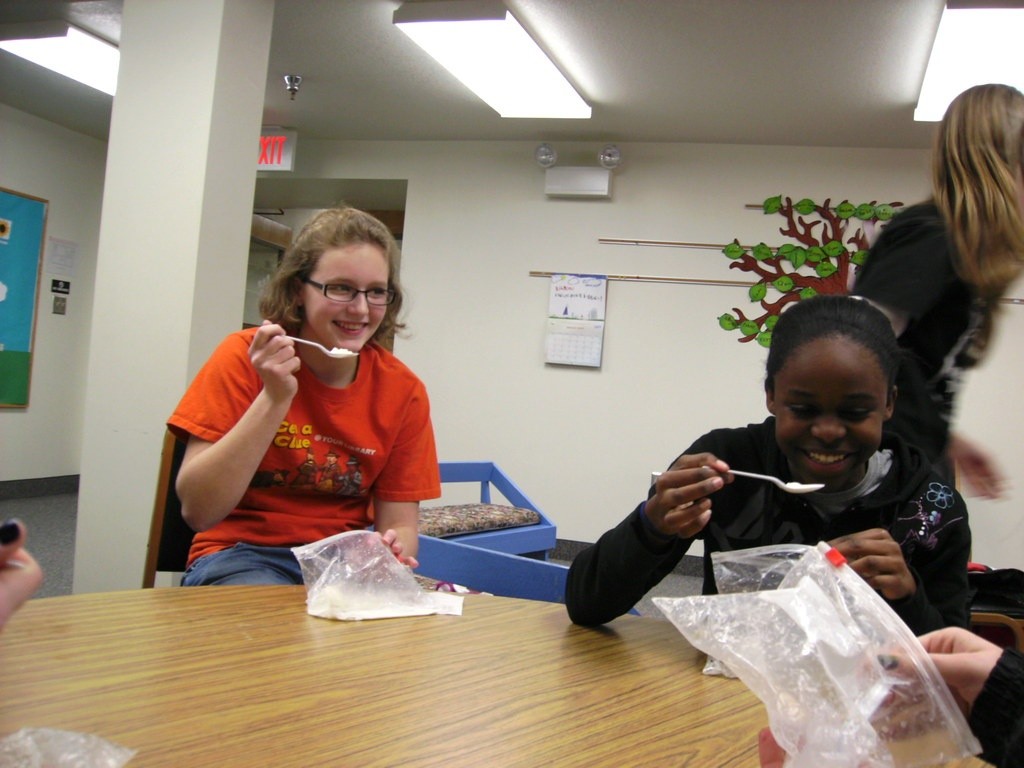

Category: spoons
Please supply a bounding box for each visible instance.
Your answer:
[282,335,359,359]
[702,465,825,494]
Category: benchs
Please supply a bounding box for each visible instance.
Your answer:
[409,460,557,566]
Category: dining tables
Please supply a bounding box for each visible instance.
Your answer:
[1,586,1007,768]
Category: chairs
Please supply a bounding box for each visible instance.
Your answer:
[966,610,1023,653]
[141,429,200,588]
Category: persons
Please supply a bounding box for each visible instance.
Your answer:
[854,79,1024,508]
[0,520,43,637]
[864,626,1024,768]
[165,205,445,588]
[565,294,970,646]
